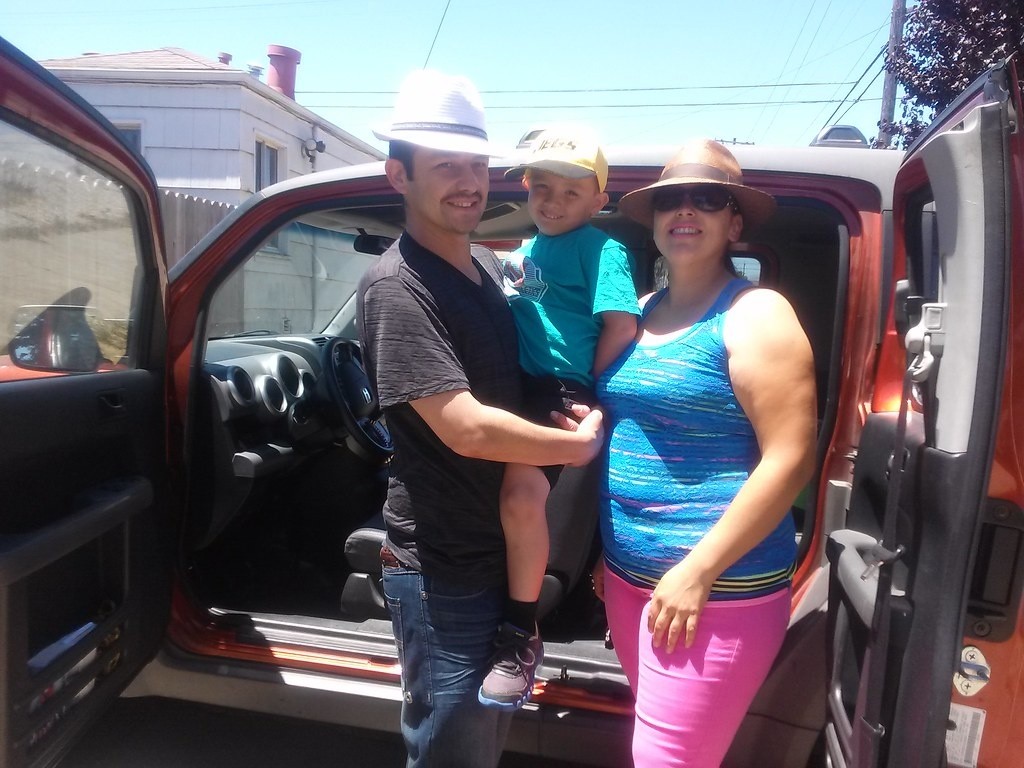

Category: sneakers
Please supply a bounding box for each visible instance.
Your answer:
[478,620,545,712]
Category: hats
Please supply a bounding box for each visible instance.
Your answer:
[372,70,512,160]
[518,126,609,193]
[617,137,778,231]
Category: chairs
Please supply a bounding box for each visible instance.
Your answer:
[338,444,607,640]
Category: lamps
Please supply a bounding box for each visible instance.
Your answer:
[304,138,326,163]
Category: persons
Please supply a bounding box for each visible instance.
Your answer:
[354,80,604,767]
[477,126,639,709]
[593,140,818,767]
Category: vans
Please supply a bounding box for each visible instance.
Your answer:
[0,37,1023,767]
